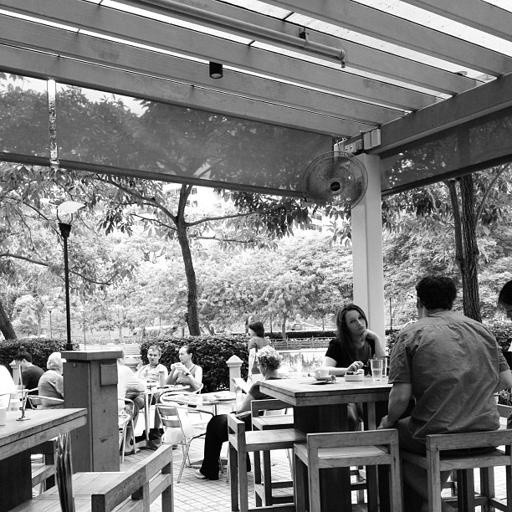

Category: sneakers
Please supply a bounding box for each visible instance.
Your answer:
[196,468,207,479]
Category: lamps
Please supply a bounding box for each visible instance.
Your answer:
[141,1,349,70]
[207,61,225,79]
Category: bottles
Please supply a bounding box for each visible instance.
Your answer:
[159,370,165,386]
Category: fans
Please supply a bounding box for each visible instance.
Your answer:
[298,146,371,215]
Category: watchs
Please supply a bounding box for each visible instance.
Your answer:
[185,371,191,376]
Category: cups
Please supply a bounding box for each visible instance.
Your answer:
[370,356,387,382]
[313,368,329,379]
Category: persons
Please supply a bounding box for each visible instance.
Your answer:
[320,304,387,431]
[126,346,169,408]
[155,344,203,429]
[15,350,44,409]
[247,322,269,382]
[377,275,512,512]
[195,344,283,480]
[104,343,146,454]
[37,351,66,407]
[497,280,512,365]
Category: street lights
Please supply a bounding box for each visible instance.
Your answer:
[52,199,87,350]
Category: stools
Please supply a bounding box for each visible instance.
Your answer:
[0,342,510,511]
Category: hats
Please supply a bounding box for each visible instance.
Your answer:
[249,322,264,337]
